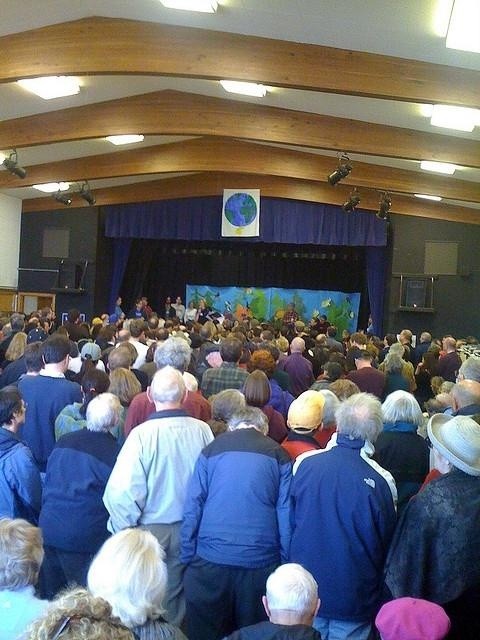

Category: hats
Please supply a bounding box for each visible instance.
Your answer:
[427,413,479,475]
[81,343,102,361]
[288,390,326,435]
[93,318,103,324]
[376,597,450,640]
[27,329,47,343]
[1,387,21,423]
[109,314,118,324]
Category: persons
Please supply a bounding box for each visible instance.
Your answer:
[1,295,479,639]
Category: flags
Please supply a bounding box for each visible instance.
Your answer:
[218,187,262,239]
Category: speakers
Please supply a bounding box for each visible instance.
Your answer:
[60,262,82,289]
[405,278,426,308]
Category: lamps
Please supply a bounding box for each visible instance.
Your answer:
[374,192,392,220]
[80,180,96,205]
[341,187,361,212]
[3,147,27,179]
[328,151,352,185]
